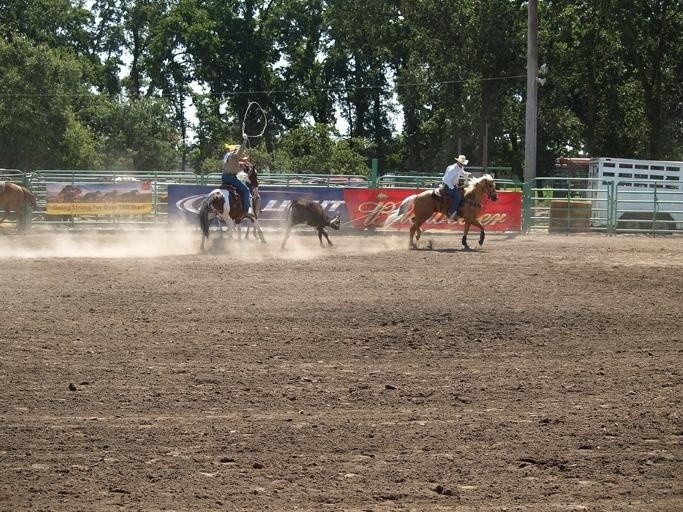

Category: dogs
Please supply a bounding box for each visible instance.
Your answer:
[81,190,102,202]
[0,181,36,234]
[382,171,498,251]
[102,189,119,200]
[158,194,168,220]
[198,161,266,254]
[121,189,139,200]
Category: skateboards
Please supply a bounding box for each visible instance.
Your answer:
[280,197,341,251]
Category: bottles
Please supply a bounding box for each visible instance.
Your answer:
[454,155,468,165]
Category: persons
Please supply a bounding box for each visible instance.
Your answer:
[440,154,472,222]
[221,133,255,217]
[488,170,500,191]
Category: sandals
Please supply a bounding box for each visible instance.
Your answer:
[553,157,592,186]
[465,165,513,178]
